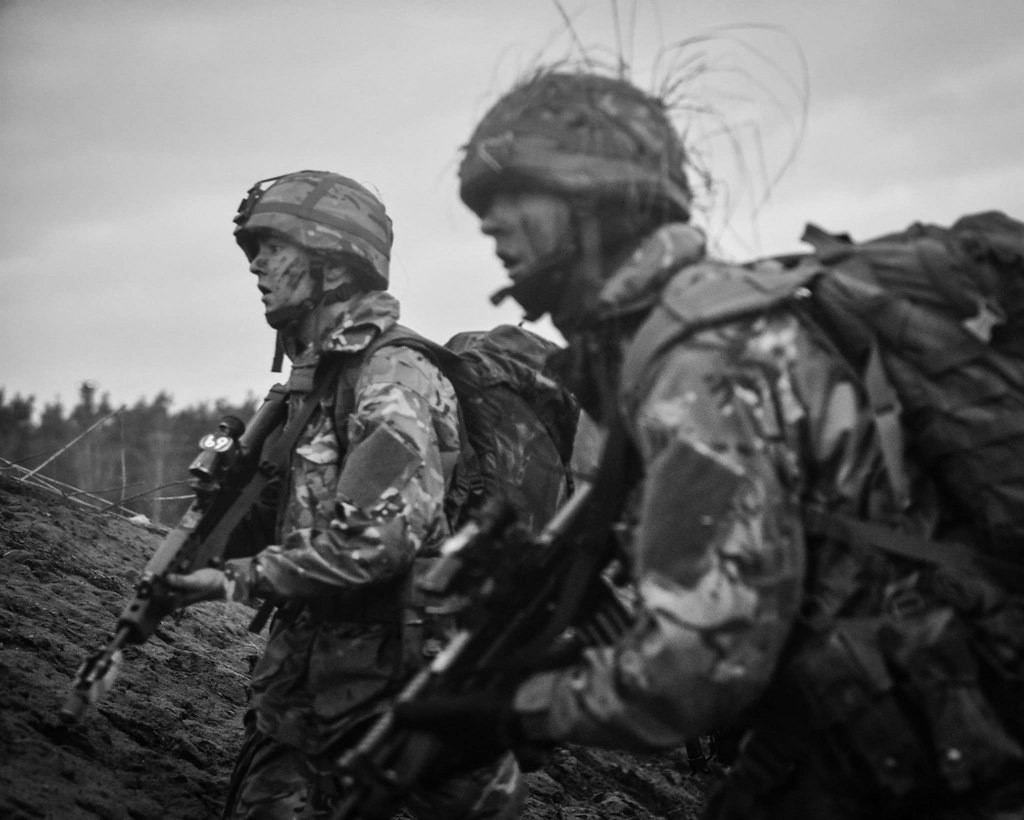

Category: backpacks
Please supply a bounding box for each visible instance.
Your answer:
[333,319,583,627]
[615,210,1024,591]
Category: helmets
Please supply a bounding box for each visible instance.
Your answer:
[232,170,395,291]
[457,73,692,222]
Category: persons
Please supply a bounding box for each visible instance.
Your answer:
[460,73,1002,820]
[159,170,471,820]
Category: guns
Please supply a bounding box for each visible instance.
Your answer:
[57,380,291,725]
[330,478,618,818]
